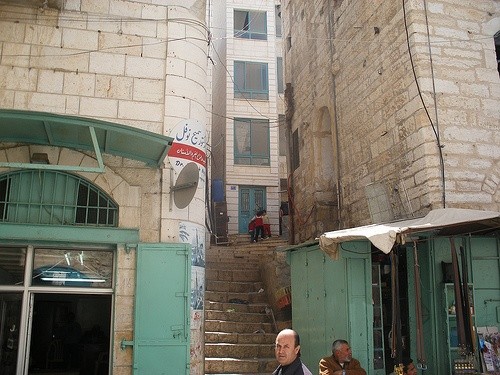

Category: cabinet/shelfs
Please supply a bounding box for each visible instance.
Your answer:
[445,283,480,375]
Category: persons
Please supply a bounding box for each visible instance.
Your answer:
[248,210,272,244]
[318,339,367,375]
[271,328,312,375]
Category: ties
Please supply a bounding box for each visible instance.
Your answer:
[278,368,282,375]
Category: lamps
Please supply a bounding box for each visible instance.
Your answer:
[79,251,84,266]
[64,250,71,265]
